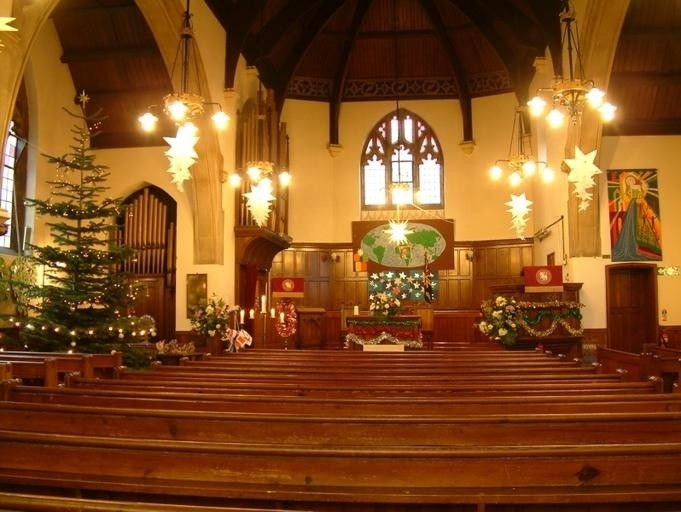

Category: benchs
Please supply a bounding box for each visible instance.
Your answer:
[0,347,681,511]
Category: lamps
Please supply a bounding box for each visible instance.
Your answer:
[138,1,294,189]
[490,0,618,186]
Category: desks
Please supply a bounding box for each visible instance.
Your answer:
[297,308,327,348]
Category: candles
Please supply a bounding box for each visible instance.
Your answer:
[241,294,285,324]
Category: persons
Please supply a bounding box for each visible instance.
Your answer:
[611,171,662,261]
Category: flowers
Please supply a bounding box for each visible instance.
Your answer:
[190,292,240,336]
[473,296,523,349]
